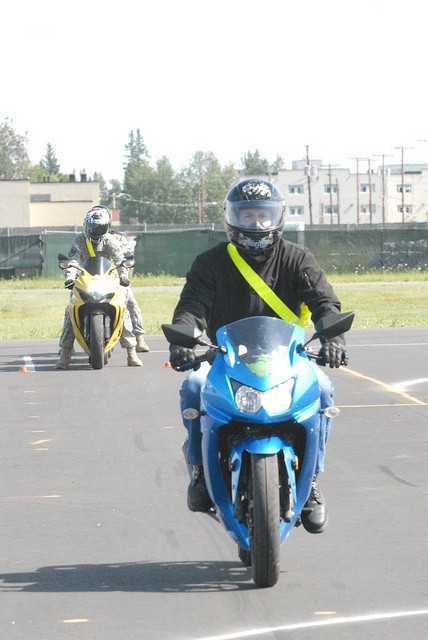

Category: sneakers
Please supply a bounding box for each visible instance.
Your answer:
[127,353,143,366]
[302,480,328,535]
[55,354,71,369]
[137,342,150,352]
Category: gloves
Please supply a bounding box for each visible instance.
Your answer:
[64,280,75,289]
[170,347,200,372]
[120,279,130,287]
[316,342,349,368]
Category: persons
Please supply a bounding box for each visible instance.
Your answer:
[169,179,349,534]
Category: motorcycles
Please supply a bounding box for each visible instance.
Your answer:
[58,256,136,369]
[161,313,354,588]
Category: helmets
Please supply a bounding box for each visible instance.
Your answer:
[224,178,286,252]
[83,208,111,244]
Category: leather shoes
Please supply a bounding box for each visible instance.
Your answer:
[187,464,212,512]
